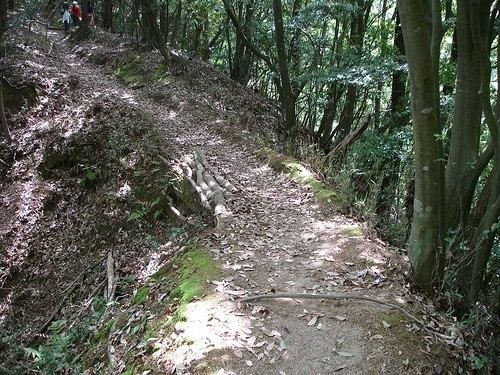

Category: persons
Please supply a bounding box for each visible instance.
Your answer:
[71,1,81,28]
[61,2,71,35]
[87,5,94,25]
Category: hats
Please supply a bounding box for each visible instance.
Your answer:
[72,1,77,4]
